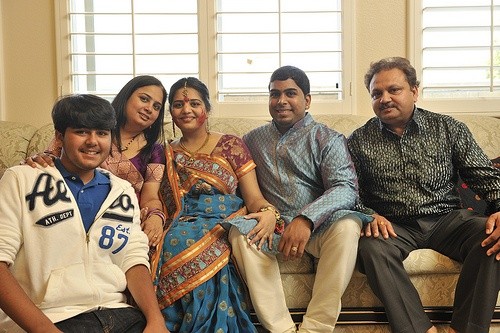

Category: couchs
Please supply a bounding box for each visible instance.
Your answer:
[0,114,500,323]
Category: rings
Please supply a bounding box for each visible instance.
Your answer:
[291,247,297,252]
[380,221,384,226]
[153,234,157,239]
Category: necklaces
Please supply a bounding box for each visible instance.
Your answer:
[193,131,209,153]
[121,132,141,151]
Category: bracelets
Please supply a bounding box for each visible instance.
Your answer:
[146,208,166,227]
[256,204,280,221]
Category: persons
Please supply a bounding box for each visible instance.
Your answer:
[216,66,375,333]
[19,75,167,247]
[347,57,500,333]
[140,77,277,333]
[0,94,170,333]
